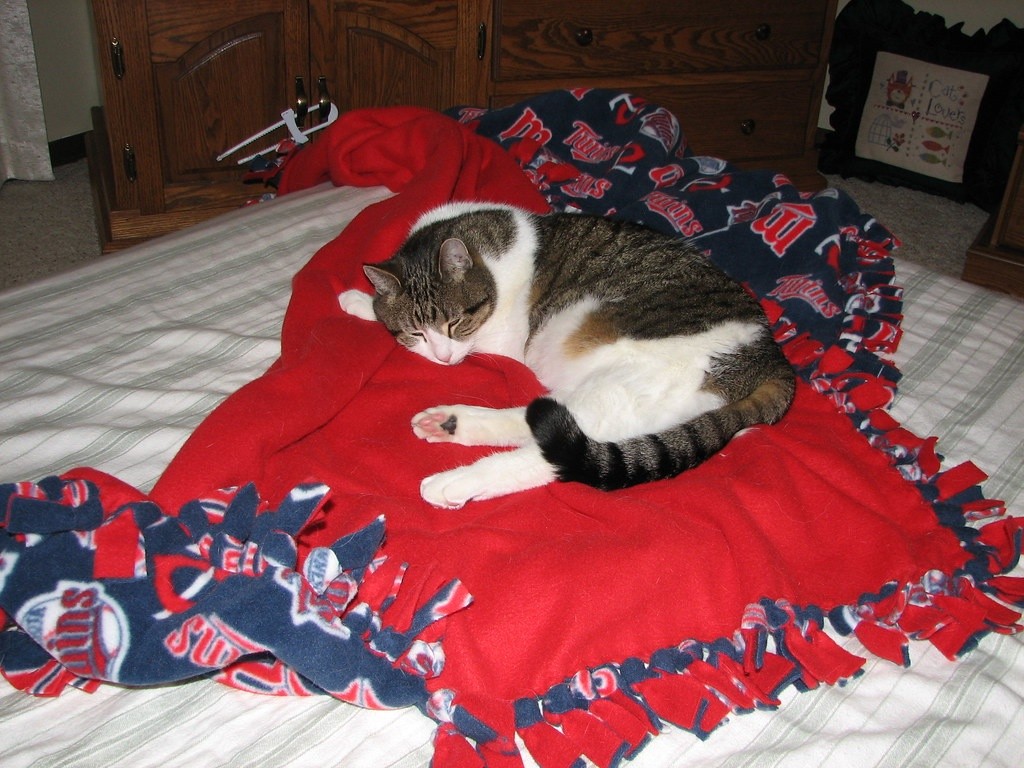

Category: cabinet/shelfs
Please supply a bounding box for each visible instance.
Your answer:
[78,0,839,258]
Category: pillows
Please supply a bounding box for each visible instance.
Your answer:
[812,0,1024,214]
[820,168,990,281]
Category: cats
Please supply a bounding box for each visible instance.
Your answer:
[336,201,797,508]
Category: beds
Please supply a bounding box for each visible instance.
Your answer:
[0,180,1024,763]
